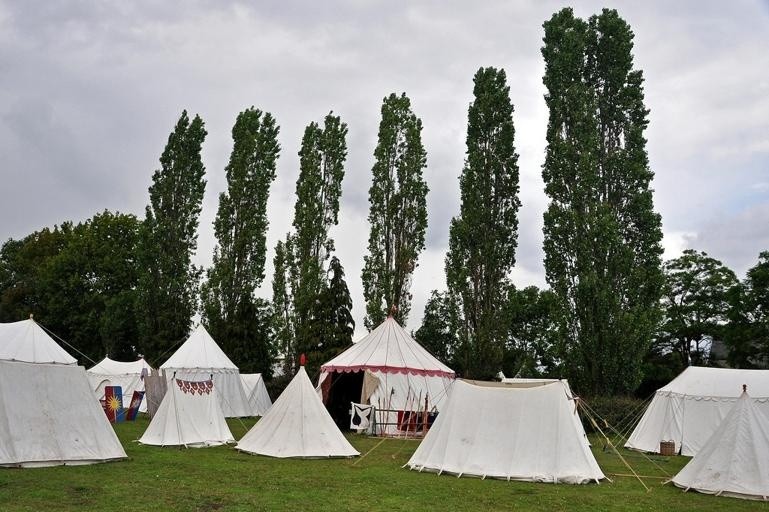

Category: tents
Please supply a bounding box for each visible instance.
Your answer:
[661,382,769,503]
[309,310,456,440]
[622,364,769,458]
[0,360,131,471]
[0,312,80,365]
[130,371,239,450]
[234,354,362,460]
[239,373,272,417]
[86,353,155,414]
[157,323,253,417]
[406,377,606,485]
